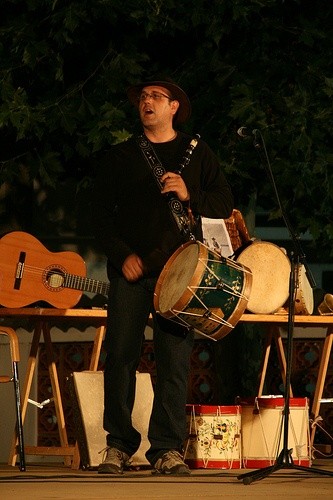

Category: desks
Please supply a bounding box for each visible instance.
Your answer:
[0,301,333,466]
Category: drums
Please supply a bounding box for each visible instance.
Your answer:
[228,239,292,315]
[234,394,312,469]
[292,261,315,316]
[150,239,253,343]
[185,403,242,470]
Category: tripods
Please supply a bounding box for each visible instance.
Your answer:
[237,130,333,486]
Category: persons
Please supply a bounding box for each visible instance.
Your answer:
[80,71,231,476]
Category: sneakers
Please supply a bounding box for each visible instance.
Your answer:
[97,445,130,473]
[151,449,192,476]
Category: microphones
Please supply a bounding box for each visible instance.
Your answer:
[236,126,260,138]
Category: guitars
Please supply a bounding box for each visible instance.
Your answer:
[0,229,111,310]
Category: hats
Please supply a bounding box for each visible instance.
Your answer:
[128,73,192,126]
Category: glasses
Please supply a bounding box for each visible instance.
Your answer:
[138,90,175,101]
